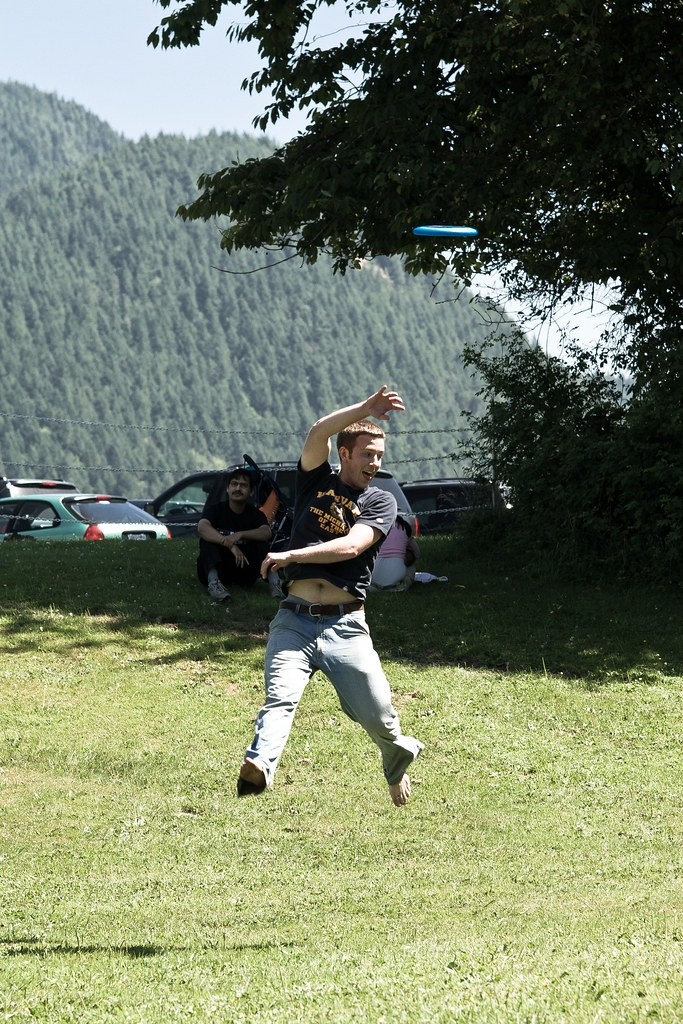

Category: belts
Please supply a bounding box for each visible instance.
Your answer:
[279,601,364,617]
[370,581,403,590]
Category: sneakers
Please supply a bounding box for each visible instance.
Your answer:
[269,580,286,599]
[208,579,231,602]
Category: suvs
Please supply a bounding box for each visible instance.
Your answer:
[141,459,420,540]
[0,475,81,528]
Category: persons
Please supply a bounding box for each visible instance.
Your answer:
[367,515,415,592]
[240,384,426,808]
[197,468,273,601]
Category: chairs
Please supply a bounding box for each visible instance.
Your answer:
[243,454,294,550]
[14,516,31,530]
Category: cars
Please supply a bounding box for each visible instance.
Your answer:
[0,494,172,543]
[128,500,205,517]
[399,478,508,536]
